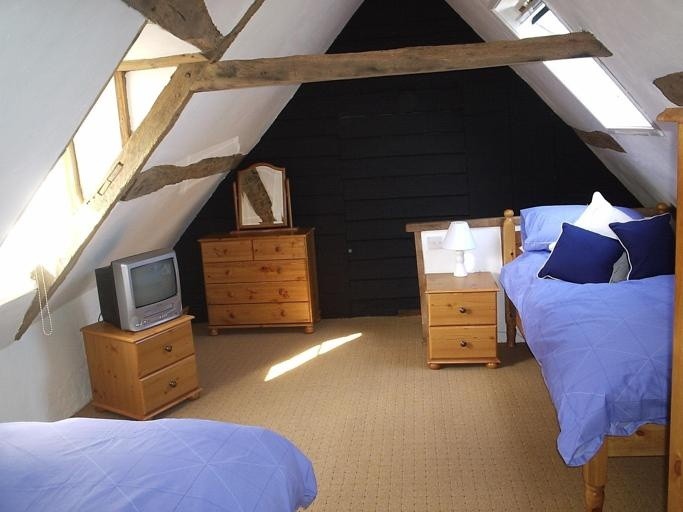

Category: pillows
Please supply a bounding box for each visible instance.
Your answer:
[533,219,626,285]
[604,208,676,282]
[516,202,646,254]
[544,190,641,284]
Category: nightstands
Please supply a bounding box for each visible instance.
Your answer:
[421,270,504,371]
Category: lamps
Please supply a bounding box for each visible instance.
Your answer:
[438,217,476,278]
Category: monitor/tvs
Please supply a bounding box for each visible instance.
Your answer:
[95,249,183,332]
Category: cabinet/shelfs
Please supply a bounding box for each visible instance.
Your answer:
[74,310,203,424]
[192,225,321,336]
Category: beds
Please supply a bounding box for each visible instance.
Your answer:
[493,198,680,512]
[0,415,319,512]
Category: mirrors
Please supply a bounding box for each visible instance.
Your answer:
[224,160,299,236]
